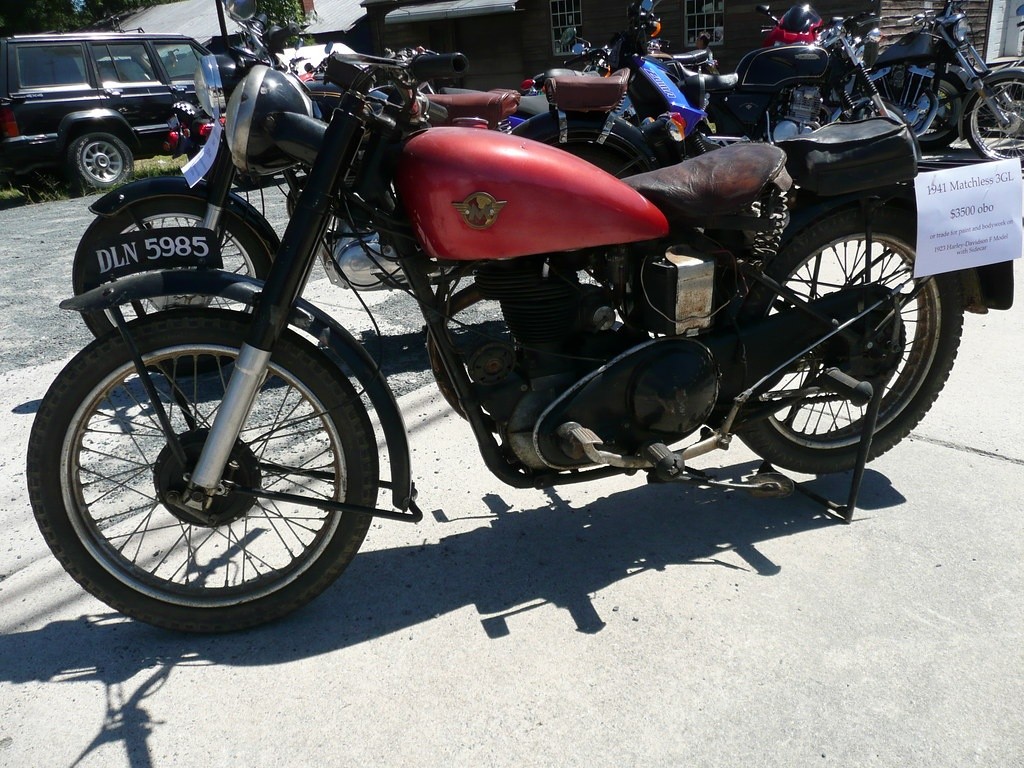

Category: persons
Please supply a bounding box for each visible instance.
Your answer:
[300,63,316,81]
[693,35,719,75]
[384,46,428,61]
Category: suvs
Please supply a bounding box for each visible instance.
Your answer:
[0,28,222,192]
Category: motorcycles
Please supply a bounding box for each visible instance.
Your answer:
[23,47,1018,635]
[228,0,1024,305]
